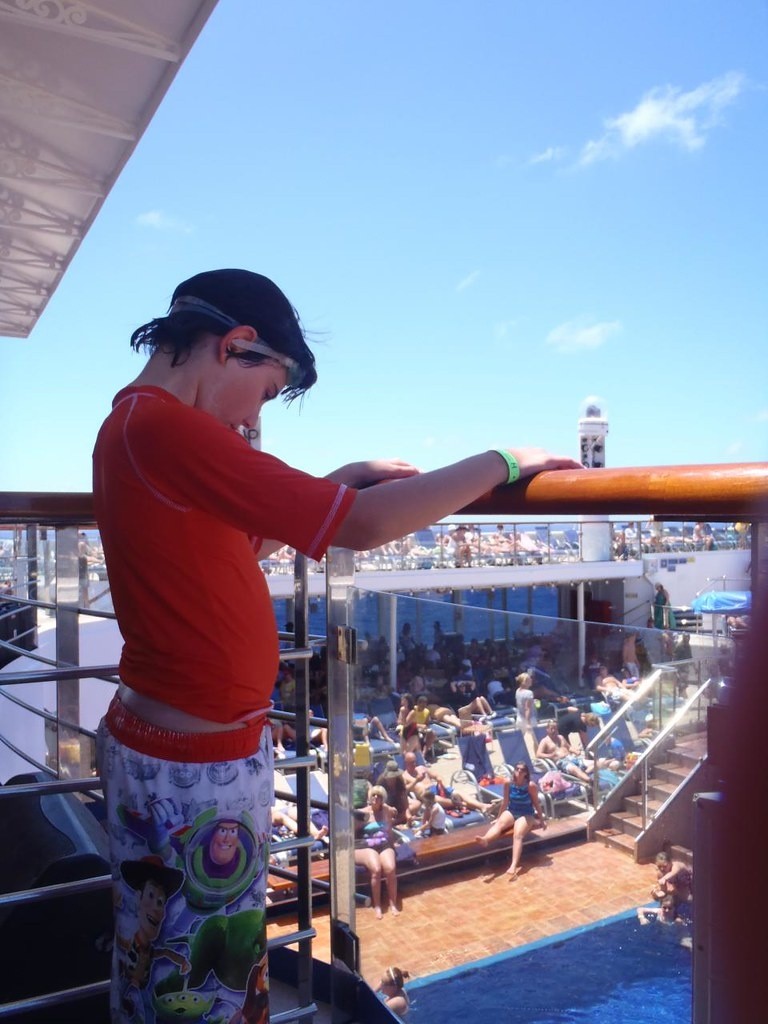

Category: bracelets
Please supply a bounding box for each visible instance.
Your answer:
[491,449,520,484]
[638,915,644,918]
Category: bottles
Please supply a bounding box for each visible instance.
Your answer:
[579,404,608,467]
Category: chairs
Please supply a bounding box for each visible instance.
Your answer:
[270,655,659,877]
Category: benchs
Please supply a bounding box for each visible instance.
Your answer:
[268,815,544,892]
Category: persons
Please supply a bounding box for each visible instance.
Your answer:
[620,630,640,676]
[637,851,693,925]
[693,522,713,551]
[78,541,105,610]
[620,522,637,559]
[735,523,751,549]
[671,633,693,699]
[357,523,554,569]
[374,967,410,1015]
[261,545,296,576]
[271,618,638,920]
[645,515,663,554]
[91,268,587,1024]
[654,582,677,630]
